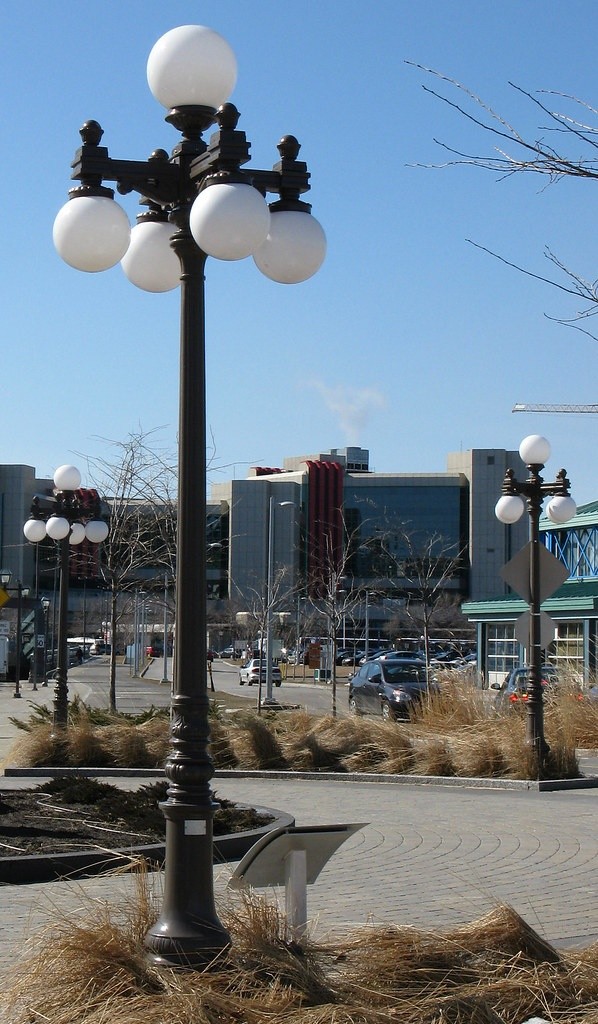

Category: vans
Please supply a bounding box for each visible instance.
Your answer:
[90,644,111,655]
[146,647,160,657]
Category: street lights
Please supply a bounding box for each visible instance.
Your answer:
[101,621,112,655]
[0,567,30,700]
[494,434,577,781]
[41,596,52,687]
[51,23,327,971]
[23,465,110,764]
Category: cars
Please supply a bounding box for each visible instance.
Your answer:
[348,658,442,722]
[68,647,89,665]
[281,646,477,681]
[247,650,265,658]
[219,647,242,658]
[491,666,588,724]
[206,649,213,662]
[239,658,282,687]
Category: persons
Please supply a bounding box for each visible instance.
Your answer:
[76,647,83,664]
[207,648,214,668]
[96,642,101,657]
